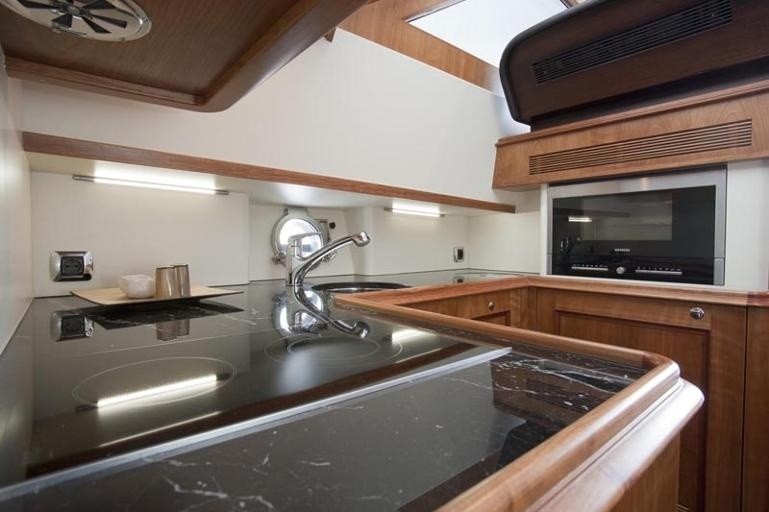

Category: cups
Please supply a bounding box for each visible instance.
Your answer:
[170,264,190,298]
[155,267,182,297]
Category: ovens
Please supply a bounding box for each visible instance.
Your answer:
[539,163,765,293]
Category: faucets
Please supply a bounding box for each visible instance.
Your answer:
[285,230,370,286]
[270,285,370,341]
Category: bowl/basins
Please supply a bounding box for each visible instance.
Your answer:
[119,274,154,297]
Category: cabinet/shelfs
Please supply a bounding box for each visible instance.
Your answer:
[1,257,769,512]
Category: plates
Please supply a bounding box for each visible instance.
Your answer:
[70,279,245,305]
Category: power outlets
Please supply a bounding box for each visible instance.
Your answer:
[48,246,95,282]
[48,304,95,343]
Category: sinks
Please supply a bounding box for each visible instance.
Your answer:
[311,282,404,294]
